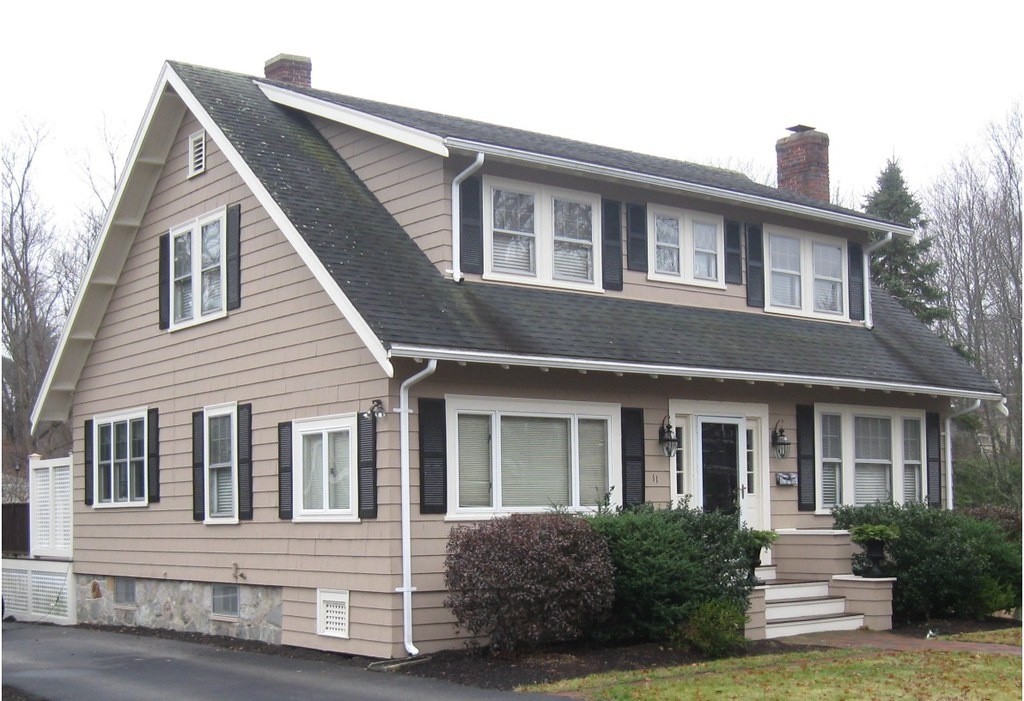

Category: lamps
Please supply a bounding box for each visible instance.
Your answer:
[659,414,679,457]
[363,399,386,421]
[772,418,791,460]
